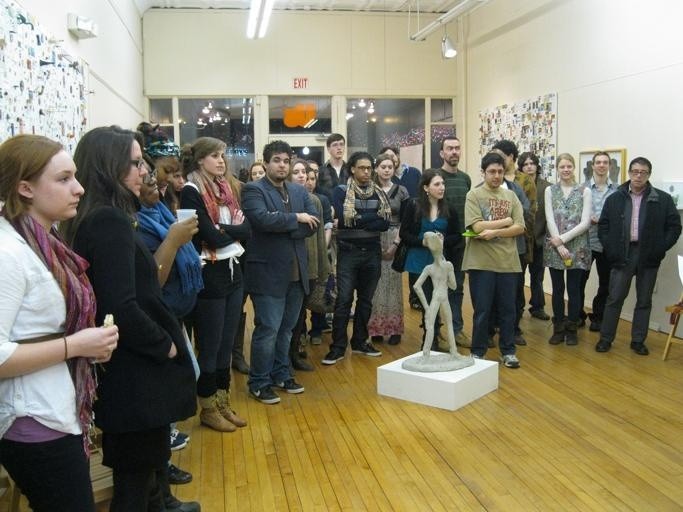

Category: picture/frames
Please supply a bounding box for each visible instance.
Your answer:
[578,148,627,186]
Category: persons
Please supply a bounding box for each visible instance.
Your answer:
[136,122,247,486]
[0,135,119,512]
[239,131,682,404]
[61,128,201,512]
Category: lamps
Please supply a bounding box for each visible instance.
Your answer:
[407,0,487,42]
[442,24,457,58]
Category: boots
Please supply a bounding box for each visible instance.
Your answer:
[198,395,236,432]
[232,312,249,374]
[216,389,247,427]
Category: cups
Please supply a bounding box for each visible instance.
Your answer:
[564,253,573,267]
[175,209,197,223]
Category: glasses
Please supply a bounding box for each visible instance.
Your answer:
[142,169,158,184]
[131,159,143,169]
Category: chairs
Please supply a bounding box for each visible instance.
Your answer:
[662,288,683,361]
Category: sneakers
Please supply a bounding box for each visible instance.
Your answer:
[168,428,200,512]
[250,311,401,404]
[412,303,649,369]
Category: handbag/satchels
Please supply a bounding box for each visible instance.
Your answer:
[306,279,338,314]
[391,242,407,274]
